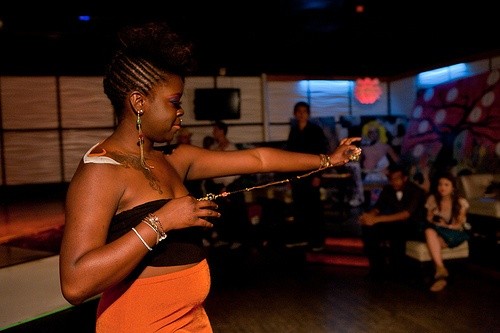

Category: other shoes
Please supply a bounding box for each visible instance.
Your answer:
[430,280,446,292]
[434,271,448,279]
[312,234,324,251]
[286,239,307,247]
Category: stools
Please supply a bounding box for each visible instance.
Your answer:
[405,241,469,298]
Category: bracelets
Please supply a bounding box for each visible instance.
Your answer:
[319,152,333,172]
[129,226,155,253]
[142,212,168,244]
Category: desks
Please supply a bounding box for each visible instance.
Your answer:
[0,254,102,333]
[0,201,66,268]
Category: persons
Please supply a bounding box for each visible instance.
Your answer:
[348,121,399,205]
[56,22,363,333]
[357,168,430,300]
[166,99,269,270]
[284,100,330,253]
[420,172,470,291]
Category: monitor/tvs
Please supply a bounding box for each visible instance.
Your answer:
[194,88,241,121]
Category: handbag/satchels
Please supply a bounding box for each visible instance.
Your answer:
[433,224,468,247]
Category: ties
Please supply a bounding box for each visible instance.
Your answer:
[396,190,402,202]
